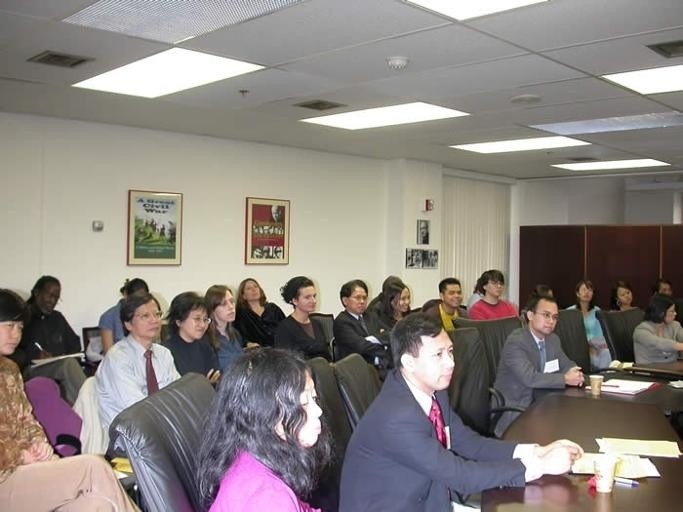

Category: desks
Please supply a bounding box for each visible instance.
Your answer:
[481,360,683,511]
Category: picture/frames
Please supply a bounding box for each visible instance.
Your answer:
[417,219,429,244]
[128,190,182,265]
[423,249,439,269]
[406,248,422,269]
[245,197,290,265]
[426,199,433,210]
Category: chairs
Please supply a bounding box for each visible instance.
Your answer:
[110,372,217,512]
[161,324,172,341]
[308,313,341,362]
[453,315,523,390]
[82,328,100,361]
[446,326,527,439]
[522,308,626,374]
[595,306,643,364]
[335,353,380,430]
[306,357,352,504]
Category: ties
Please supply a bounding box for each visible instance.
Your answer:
[428,396,447,448]
[539,342,546,374]
[144,350,159,397]
[358,316,370,337]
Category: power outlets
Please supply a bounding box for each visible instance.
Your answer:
[93,220,103,232]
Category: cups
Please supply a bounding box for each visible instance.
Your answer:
[592,443,616,495]
[586,372,604,400]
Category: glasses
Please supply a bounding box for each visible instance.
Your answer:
[45,293,62,304]
[537,312,559,321]
[133,311,163,320]
[188,316,212,324]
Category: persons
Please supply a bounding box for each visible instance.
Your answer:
[334,279,390,369]
[263,248,272,258]
[271,205,281,223]
[420,222,429,244]
[489,294,586,439]
[368,275,402,308]
[195,346,337,512]
[652,277,674,300]
[524,284,554,312]
[161,291,222,390]
[274,276,333,362]
[467,270,518,320]
[94,293,182,452]
[339,312,584,511]
[609,281,636,311]
[231,278,287,347]
[368,281,411,340]
[253,248,262,259]
[567,280,612,368]
[0,287,143,512]
[425,278,468,331]
[7,275,87,407]
[274,247,282,259]
[204,285,244,370]
[632,292,683,365]
[97,278,149,358]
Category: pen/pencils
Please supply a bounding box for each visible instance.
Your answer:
[34,342,43,351]
[615,477,640,485]
[601,384,619,387]
[615,482,639,488]
